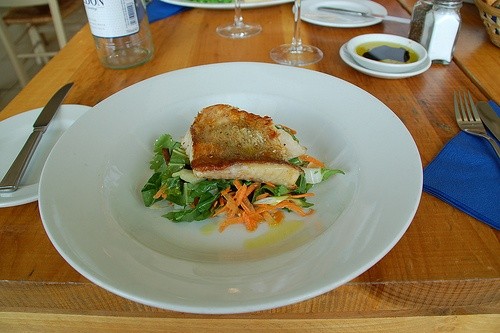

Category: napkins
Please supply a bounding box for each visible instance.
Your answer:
[146,0,193,23]
[423,99,500,229]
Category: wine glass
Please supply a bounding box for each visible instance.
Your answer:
[269,0,324,67]
[216,0,263,39]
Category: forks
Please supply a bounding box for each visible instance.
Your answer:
[453,89,500,160]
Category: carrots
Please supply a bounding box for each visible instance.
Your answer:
[210,154,326,231]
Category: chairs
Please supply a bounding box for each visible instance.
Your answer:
[0,0,84,88]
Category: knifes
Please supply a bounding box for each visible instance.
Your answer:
[0,82,75,193]
[318,6,412,25]
[477,100,500,142]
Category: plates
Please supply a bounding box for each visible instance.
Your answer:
[347,33,428,72]
[0,104,93,209]
[291,0,388,28]
[338,41,433,80]
[37,60,424,316]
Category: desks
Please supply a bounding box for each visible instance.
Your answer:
[1,0,500,332]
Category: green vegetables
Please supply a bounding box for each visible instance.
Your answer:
[142,133,346,223]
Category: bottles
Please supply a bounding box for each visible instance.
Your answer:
[408,0,436,44]
[419,0,463,66]
[83,0,154,69]
